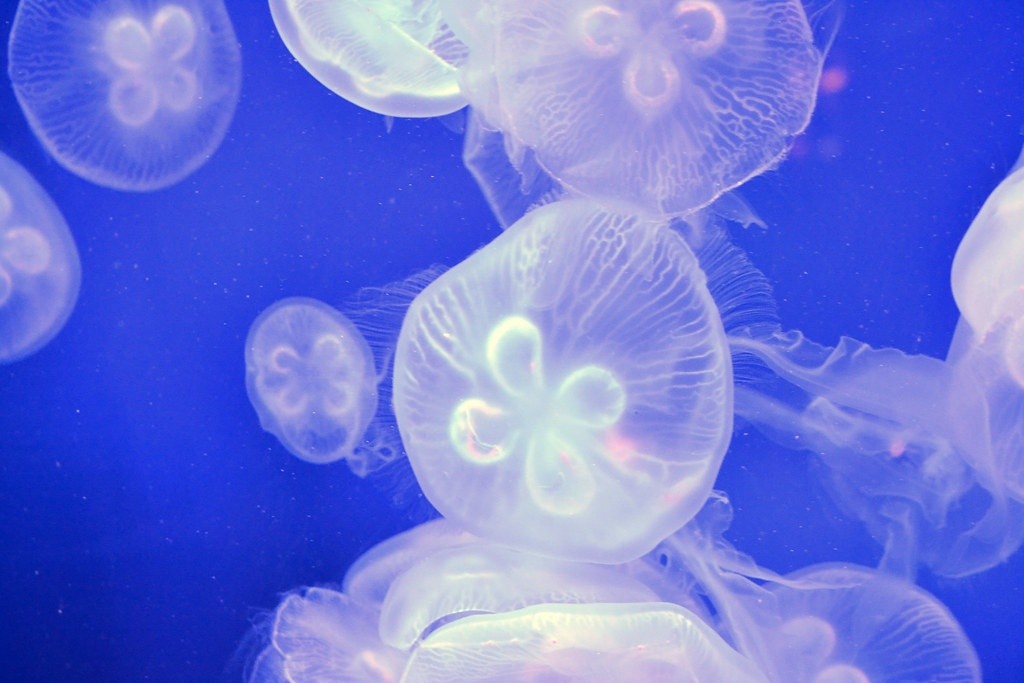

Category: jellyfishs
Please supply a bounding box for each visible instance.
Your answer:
[241,1,1024,683]
[1,153,84,369]
[8,1,242,191]
